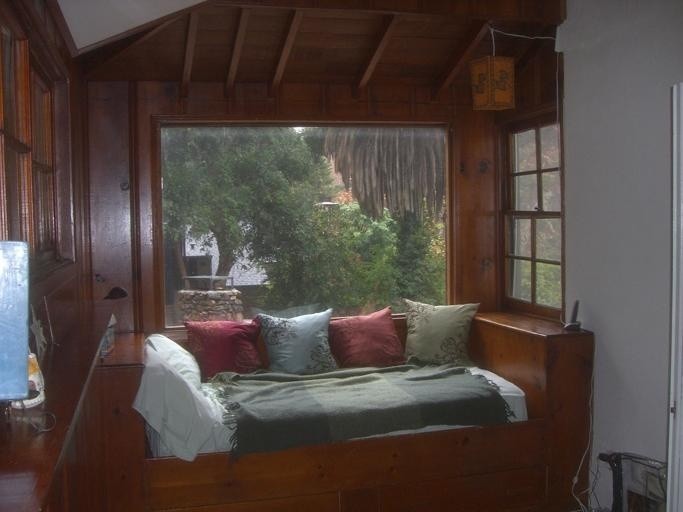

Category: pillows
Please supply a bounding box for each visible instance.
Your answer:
[402,297,481,366]
[257,308,338,374]
[184,320,262,382]
[328,305,405,366]
[133,335,214,461]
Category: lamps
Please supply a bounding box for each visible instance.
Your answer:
[0,241,30,400]
[469,28,515,111]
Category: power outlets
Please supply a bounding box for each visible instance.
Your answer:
[599,451,609,463]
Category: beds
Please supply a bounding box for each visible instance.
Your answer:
[80,309,594,512]
